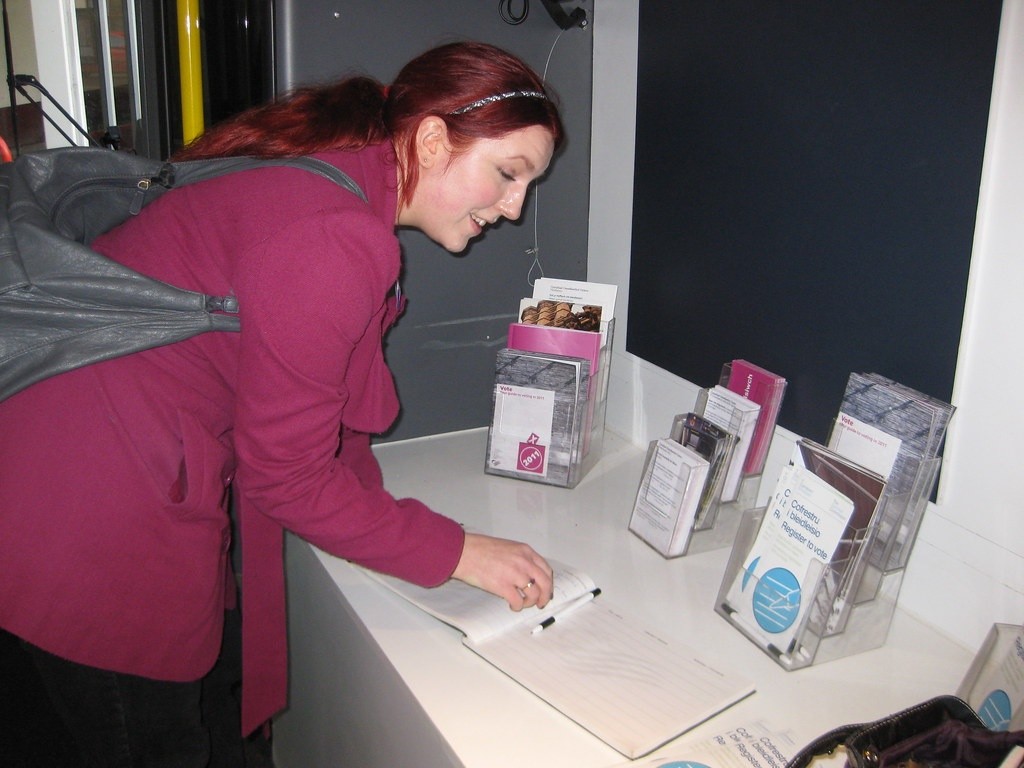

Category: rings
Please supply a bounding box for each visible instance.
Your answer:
[521,578,535,592]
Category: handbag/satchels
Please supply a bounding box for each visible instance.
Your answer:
[0,146,368,402]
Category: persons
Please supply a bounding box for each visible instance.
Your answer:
[0,40,569,768]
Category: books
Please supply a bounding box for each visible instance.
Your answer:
[484,276,618,486]
[356,518,758,762]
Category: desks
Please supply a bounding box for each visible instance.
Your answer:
[272,427,976,768]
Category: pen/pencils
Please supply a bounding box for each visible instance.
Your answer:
[530,587,602,636]
